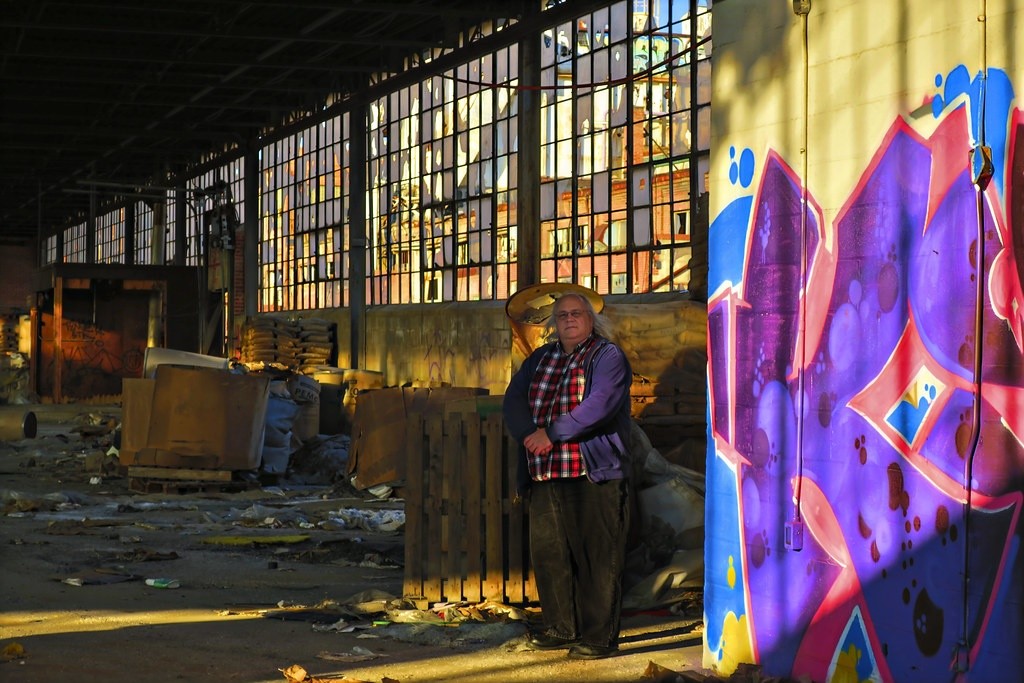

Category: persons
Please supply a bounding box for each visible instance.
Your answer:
[502,292,633,660]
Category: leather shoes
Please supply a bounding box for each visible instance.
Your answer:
[567,643,619,659]
[528,634,577,649]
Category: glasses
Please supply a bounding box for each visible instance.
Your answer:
[558,309,588,320]
